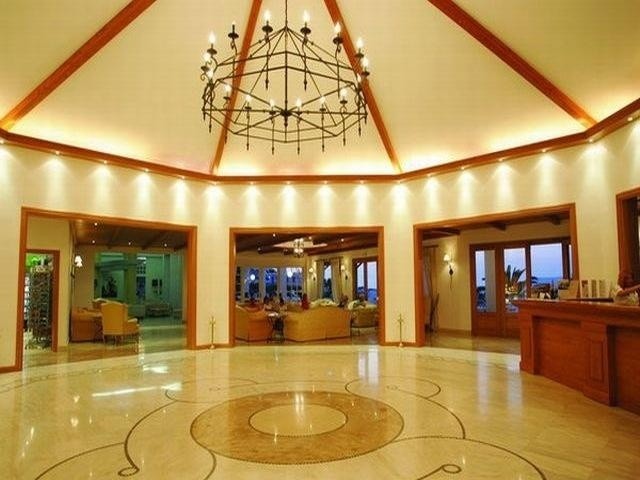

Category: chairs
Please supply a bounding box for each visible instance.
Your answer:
[100,301,142,346]
[92,299,109,309]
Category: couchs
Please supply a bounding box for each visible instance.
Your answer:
[234,298,387,343]
[69,305,104,342]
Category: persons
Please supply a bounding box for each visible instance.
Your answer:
[249,293,368,313]
[103,277,115,297]
[617,269,640,298]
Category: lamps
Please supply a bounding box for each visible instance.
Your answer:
[292,234,305,257]
[442,249,459,289]
[197,1,371,157]
[71,254,83,276]
